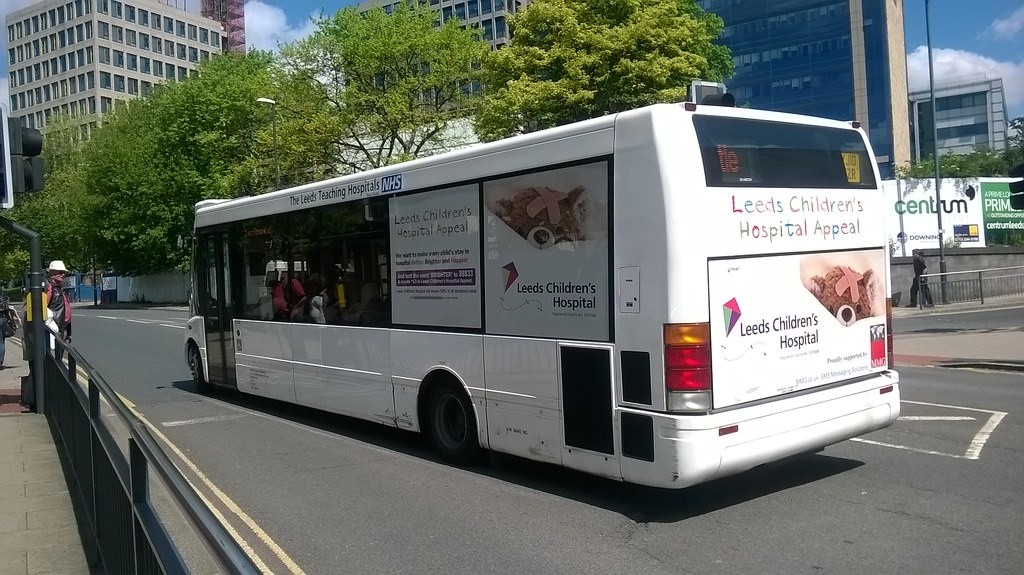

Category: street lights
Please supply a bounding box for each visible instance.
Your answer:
[256,97,280,191]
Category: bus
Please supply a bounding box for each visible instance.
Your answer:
[185,101,902,489]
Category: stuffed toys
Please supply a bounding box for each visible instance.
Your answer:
[497,185,591,249]
[811,265,880,327]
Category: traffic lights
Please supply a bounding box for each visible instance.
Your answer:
[691,80,735,107]
[1007,163,1024,210]
[0,105,47,209]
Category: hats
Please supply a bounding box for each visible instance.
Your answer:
[45,260,70,274]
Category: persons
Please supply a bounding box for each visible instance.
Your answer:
[0,298,6,370]
[273,264,380,325]
[906,249,934,308]
[23,277,60,412]
[45,261,71,331]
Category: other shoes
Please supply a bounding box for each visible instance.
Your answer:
[906,304,918,307]
[924,304,934,307]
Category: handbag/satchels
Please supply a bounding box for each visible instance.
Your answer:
[3,315,16,337]
